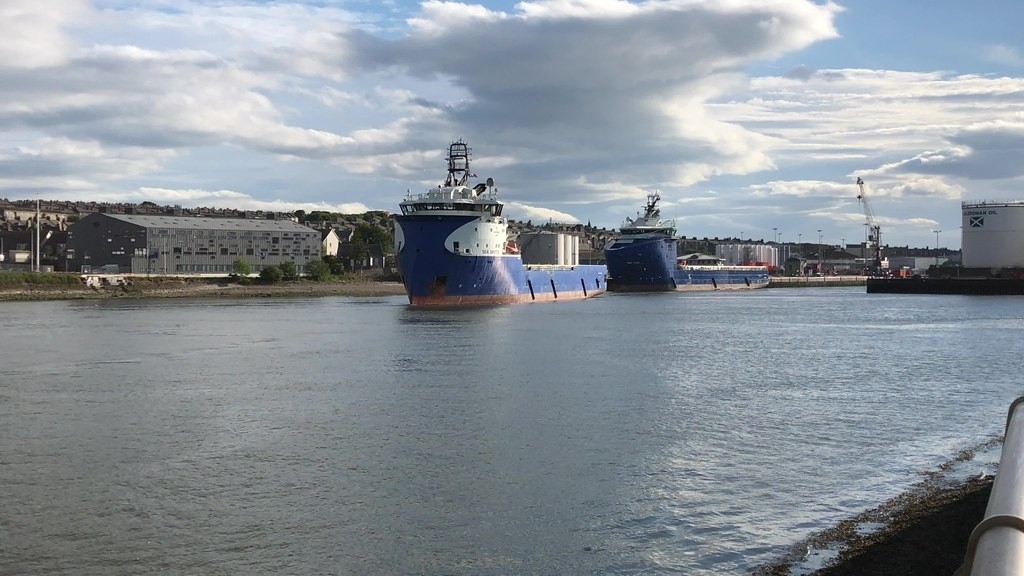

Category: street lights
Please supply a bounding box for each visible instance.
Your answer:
[817,230,825,265]
[772,228,778,243]
[798,233,802,257]
[36,191,43,274]
[777,232,783,245]
[933,230,942,267]
[841,239,846,271]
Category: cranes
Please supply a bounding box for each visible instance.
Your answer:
[856,176,883,257]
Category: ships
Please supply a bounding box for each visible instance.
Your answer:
[605,190,771,292]
[392,137,608,307]
